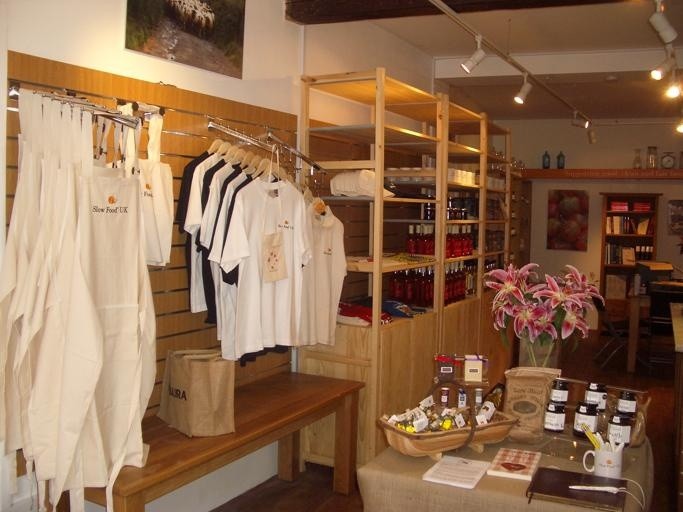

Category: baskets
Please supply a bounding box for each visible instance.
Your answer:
[378,378,520,461]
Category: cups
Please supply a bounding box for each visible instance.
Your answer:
[583,444,623,480]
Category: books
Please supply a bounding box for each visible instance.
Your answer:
[486,447,542,481]
[605,200,654,265]
[422,455,491,490]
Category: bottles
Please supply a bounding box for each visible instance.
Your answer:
[388,186,506,308]
[489,146,527,171]
[543,151,550,169]
[440,382,505,423]
[647,146,658,169]
[557,151,565,169]
[632,149,642,169]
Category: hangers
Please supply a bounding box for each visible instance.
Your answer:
[207,121,328,214]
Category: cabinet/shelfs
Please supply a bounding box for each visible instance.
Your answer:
[600,192,664,322]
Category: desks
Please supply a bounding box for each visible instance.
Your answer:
[357,401,655,512]
[38,371,366,512]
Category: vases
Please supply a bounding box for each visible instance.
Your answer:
[504,328,561,368]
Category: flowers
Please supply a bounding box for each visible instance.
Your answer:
[482,263,605,367]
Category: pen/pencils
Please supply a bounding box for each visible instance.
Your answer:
[582,424,601,449]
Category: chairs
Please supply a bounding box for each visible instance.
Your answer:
[644,285,683,376]
[591,294,650,370]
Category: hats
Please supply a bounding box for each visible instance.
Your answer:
[330,170,407,198]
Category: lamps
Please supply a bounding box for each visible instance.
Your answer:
[430,0,683,130]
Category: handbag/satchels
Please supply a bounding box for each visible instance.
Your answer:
[157,348,236,438]
[106,157,174,267]
[258,233,287,283]
